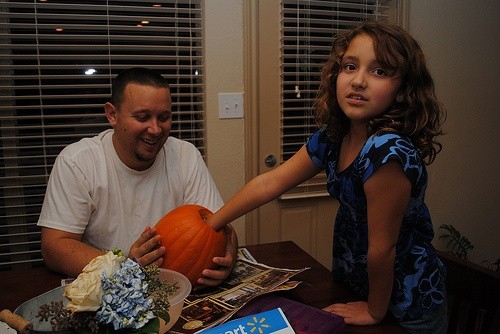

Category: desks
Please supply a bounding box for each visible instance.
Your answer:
[0,241,413,334]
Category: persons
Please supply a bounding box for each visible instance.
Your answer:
[37,67,238,291]
[206,21,447,334]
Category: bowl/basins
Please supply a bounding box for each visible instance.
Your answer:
[149,269,192,334]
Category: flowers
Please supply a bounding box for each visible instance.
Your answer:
[35,247,180,334]
